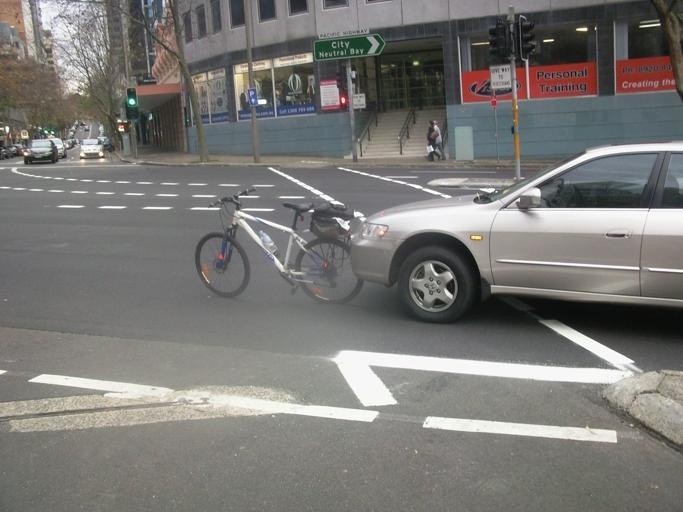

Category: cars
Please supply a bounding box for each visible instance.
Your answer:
[348,139,682,329]
[0,120,113,164]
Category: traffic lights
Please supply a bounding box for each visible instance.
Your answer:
[339,96,346,109]
[126,86,138,108]
[518,15,536,63]
[488,21,511,57]
[335,71,343,90]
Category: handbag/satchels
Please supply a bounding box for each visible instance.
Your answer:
[430,131,439,140]
[309,204,353,238]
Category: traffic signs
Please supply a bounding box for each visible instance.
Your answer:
[312,34,385,62]
[489,65,512,91]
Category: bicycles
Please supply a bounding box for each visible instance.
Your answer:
[193,184,364,307]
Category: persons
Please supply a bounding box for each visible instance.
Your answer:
[424,121,446,161]
[426,120,440,161]
[308,78,314,100]
